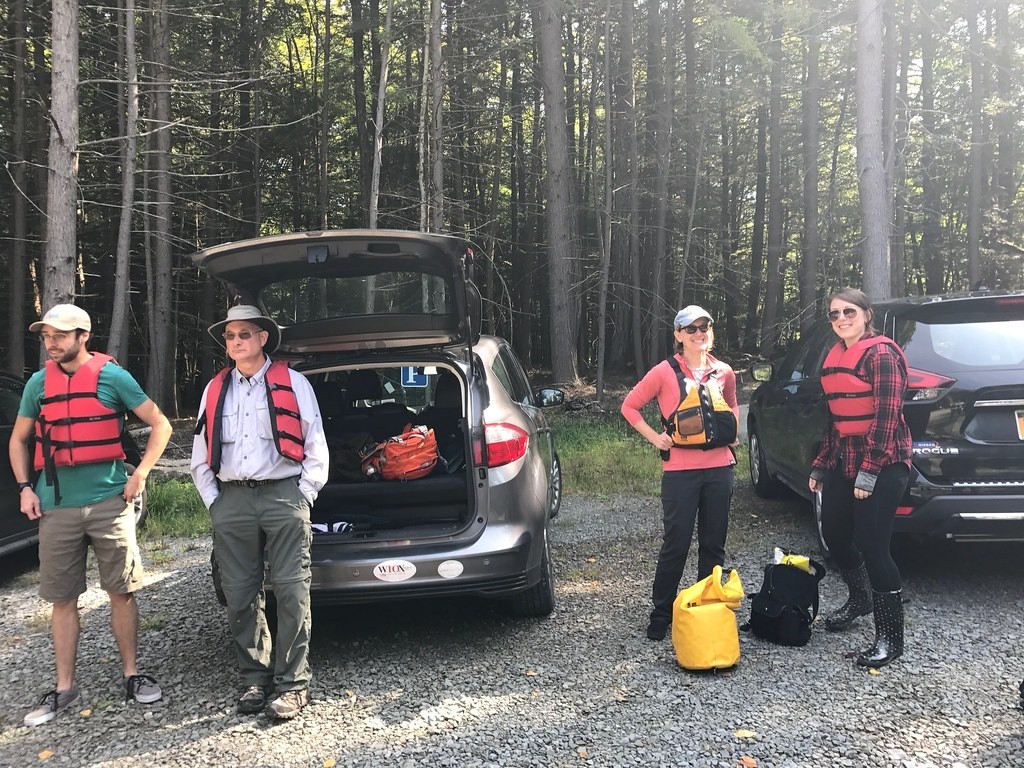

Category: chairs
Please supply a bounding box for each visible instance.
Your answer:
[315,370,463,445]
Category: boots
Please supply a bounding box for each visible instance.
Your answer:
[857,586,904,667]
[826,561,874,631]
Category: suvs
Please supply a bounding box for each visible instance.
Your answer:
[747,290,1024,572]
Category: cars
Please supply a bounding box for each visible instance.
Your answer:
[0,370,147,561]
[210,228,564,620]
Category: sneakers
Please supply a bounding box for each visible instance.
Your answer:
[122,674,162,703]
[265,688,307,719]
[24,684,81,726]
[238,685,266,714]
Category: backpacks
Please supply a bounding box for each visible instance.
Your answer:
[740,546,826,647]
[357,423,438,483]
[672,565,746,674]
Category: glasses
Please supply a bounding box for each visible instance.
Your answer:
[680,324,709,334]
[222,330,267,340]
[38,331,77,341]
[827,308,858,322]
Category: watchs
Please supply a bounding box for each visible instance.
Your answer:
[16,482,33,493]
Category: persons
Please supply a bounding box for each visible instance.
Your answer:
[8,304,173,726]
[190,305,329,720]
[809,288,913,667]
[621,305,740,640]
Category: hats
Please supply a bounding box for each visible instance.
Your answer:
[207,305,281,355]
[674,305,714,328]
[28,304,92,332]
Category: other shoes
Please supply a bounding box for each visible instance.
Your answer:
[647,620,667,641]
